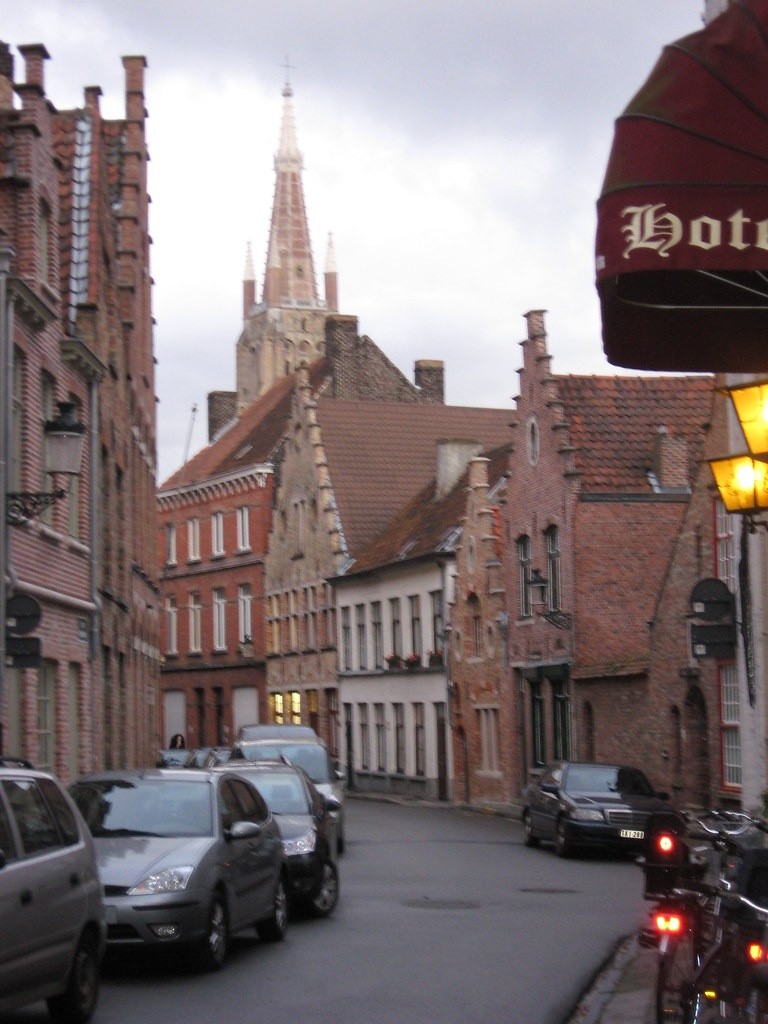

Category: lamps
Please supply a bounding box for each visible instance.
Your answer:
[5,401,89,526]
[708,451,768,534]
[723,379,768,457]
[525,568,569,630]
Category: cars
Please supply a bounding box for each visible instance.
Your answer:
[52,768,291,978]
[519,761,688,859]
[161,723,346,916]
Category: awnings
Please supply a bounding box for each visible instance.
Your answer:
[595,0,768,373]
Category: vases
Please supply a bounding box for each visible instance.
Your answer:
[407,663,420,668]
[429,659,443,666]
[389,664,401,670]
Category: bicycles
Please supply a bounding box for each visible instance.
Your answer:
[637,809,768,1024]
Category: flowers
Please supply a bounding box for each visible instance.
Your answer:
[404,653,420,664]
[385,655,402,663]
[426,649,443,659]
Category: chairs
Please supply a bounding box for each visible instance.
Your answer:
[269,785,294,810]
[292,749,315,775]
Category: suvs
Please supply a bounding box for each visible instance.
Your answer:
[0,756,110,1024]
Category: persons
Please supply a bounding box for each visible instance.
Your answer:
[169,734,185,749]
